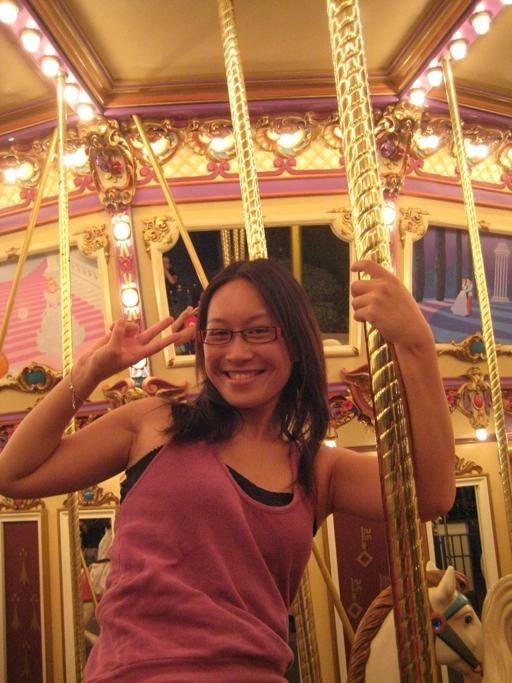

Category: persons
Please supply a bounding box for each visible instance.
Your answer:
[450,275,473,316]
[0,259,456,683]
[163,255,178,308]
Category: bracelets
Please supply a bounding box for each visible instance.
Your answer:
[68,366,91,404]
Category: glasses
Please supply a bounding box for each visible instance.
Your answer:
[200,327,282,346]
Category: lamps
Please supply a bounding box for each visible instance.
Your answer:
[0,2,95,122]
[121,281,142,322]
[110,212,134,248]
[406,1,511,108]
